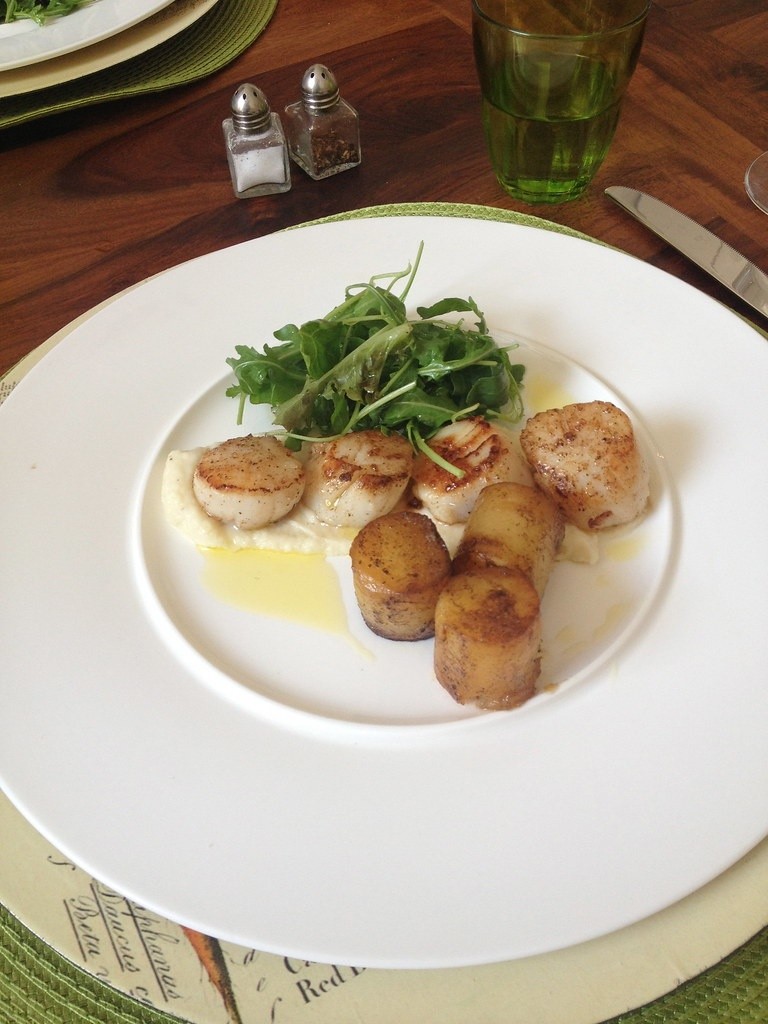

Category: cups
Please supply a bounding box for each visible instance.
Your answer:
[470,0,654,204]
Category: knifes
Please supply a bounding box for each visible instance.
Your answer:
[604,186,768,318]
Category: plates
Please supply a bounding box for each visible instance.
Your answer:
[0,0,176,74]
[0,215,768,970]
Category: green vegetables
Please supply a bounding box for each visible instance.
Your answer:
[0,0,98,26]
[224,240,527,477]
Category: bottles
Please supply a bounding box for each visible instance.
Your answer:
[221,82,291,198]
[284,64,362,180]
